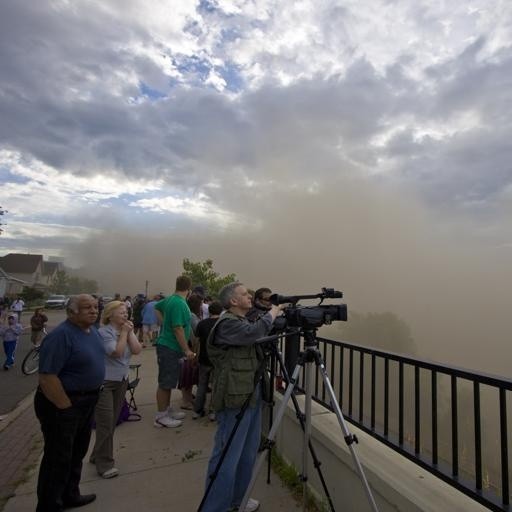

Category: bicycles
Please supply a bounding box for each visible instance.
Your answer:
[21,326,50,376]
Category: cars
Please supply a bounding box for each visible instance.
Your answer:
[45,293,115,309]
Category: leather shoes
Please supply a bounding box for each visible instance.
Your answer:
[71,493,97,508]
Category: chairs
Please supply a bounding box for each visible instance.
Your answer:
[122,362,142,413]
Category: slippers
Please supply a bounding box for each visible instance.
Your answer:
[181,404,194,410]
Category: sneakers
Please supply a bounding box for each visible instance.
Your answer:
[167,407,186,418]
[96,468,121,479]
[154,413,184,427]
[191,412,205,419]
[208,413,217,421]
[242,496,260,511]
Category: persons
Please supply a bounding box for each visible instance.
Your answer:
[12,297,24,323]
[89,300,141,479]
[154,276,284,428]
[33,293,105,511]
[199,281,282,512]
[92,293,164,349]
[1,315,23,370]
[31,308,48,344]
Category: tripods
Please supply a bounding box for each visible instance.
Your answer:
[239,349,381,512]
[195,346,336,512]
